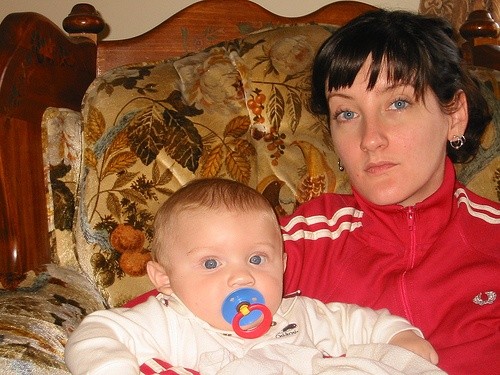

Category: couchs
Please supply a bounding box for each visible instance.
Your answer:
[0,0,500,375]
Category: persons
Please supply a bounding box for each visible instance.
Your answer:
[66,177,439,375]
[123,8,500,375]
